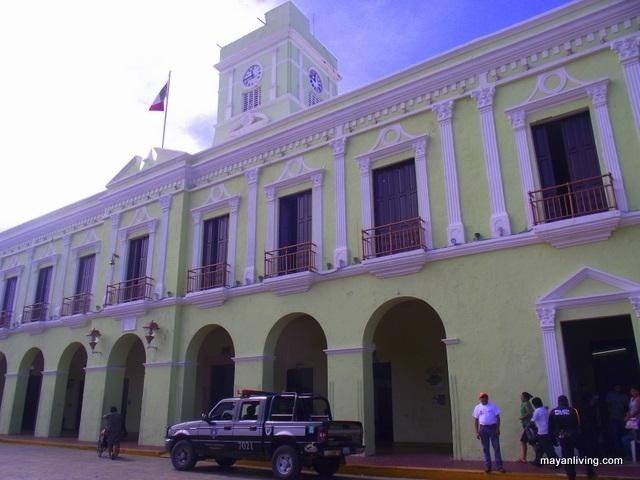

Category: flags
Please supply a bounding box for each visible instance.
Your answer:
[148,81,168,111]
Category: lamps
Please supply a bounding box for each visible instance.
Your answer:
[142,319,160,350]
[86,328,102,354]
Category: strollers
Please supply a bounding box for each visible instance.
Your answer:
[95,425,124,459]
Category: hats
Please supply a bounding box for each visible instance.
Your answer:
[480,392,488,398]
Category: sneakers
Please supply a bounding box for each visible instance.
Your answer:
[518,457,562,470]
[485,466,505,472]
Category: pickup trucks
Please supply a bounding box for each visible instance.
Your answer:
[167,388,367,479]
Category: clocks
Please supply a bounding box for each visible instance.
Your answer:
[308,67,323,95]
[242,63,263,89]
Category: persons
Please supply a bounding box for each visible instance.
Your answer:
[623,387,640,463]
[472,392,507,474]
[516,391,535,465]
[242,405,257,420]
[606,383,629,458]
[101,406,125,460]
[548,395,599,480]
[521,397,562,471]
